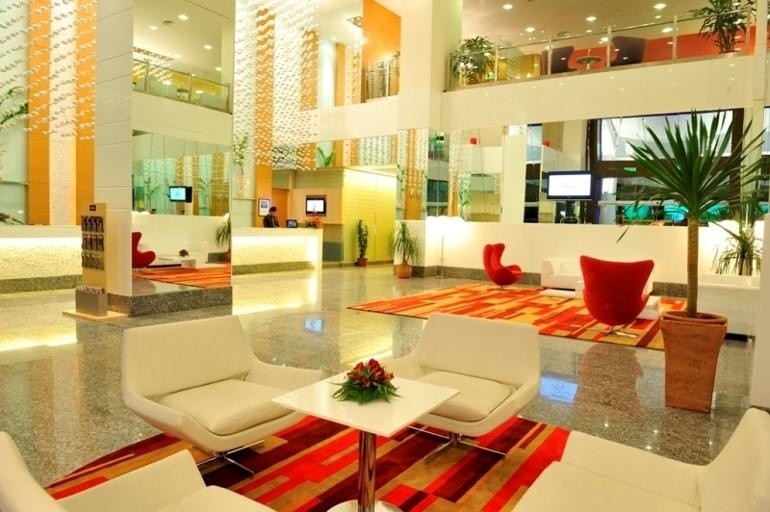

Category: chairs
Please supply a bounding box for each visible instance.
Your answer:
[508,404,769,512]
[0,430,276,512]
[605,36,646,66]
[371,310,543,466]
[542,46,578,75]
[119,313,325,481]
[132,227,157,273]
[482,242,524,296]
[580,255,655,339]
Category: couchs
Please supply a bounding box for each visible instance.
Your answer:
[692,270,760,340]
[540,255,657,294]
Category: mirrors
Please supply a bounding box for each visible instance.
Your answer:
[131,0,234,294]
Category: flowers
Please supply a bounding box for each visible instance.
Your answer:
[325,357,401,406]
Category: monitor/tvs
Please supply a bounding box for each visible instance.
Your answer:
[168,185,188,201]
[286,220,297,227]
[305,197,325,214]
[538,372,579,403]
[622,203,769,223]
[303,317,325,333]
[546,170,592,200]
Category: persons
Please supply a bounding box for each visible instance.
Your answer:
[263,206,280,228]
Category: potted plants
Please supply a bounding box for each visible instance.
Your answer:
[215,211,231,264]
[356,219,368,267]
[387,220,419,279]
[609,103,769,414]
[685,1,757,54]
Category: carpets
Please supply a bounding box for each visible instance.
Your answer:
[134,263,231,291]
[346,279,689,353]
[42,412,569,511]
[131,265,231,289]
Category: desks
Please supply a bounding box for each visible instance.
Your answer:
[271,367,461,511]
[576,56,602,71]
